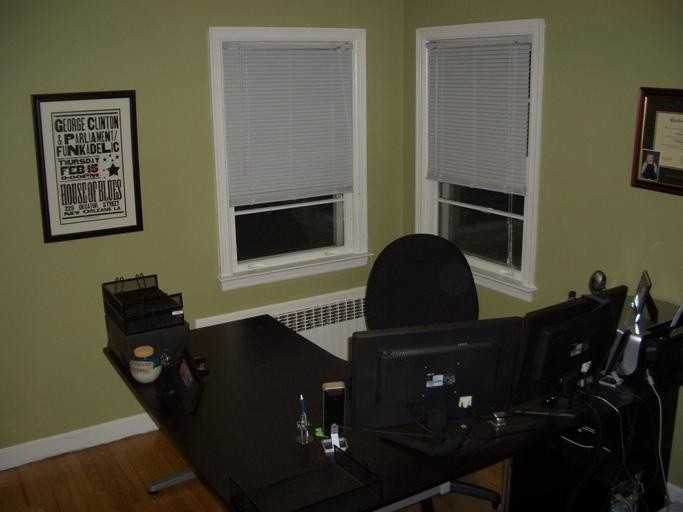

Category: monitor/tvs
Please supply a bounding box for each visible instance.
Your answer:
[349,316,524,464]
[520,286,629,402]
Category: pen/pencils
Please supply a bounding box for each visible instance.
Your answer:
[300,394,305,415]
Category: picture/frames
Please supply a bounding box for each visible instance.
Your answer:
[32,90,144,244]
[632,85,683,197]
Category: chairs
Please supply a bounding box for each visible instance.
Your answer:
[364,235,502,511]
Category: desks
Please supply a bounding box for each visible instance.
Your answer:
[102,297,682,512]
